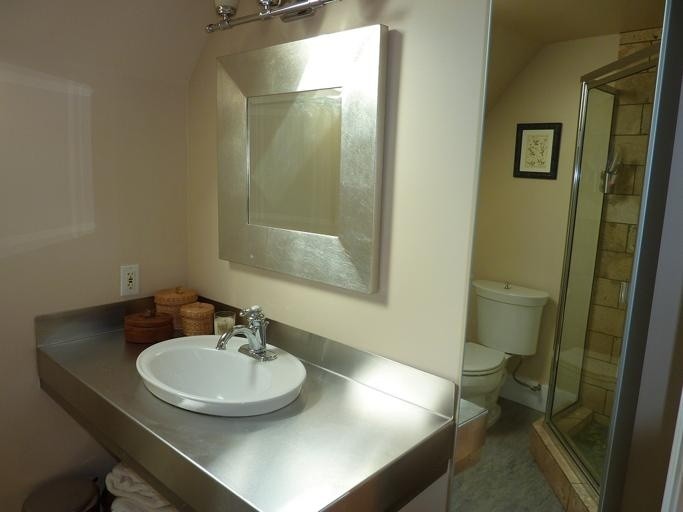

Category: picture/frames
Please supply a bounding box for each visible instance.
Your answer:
[513,122,560,179]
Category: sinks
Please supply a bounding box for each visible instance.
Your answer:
[135,334,308,417]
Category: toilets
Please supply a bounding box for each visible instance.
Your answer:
[461,279,550,428]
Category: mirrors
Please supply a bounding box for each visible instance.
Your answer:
[216,23,387,295]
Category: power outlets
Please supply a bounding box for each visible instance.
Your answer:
[120,264,140,296]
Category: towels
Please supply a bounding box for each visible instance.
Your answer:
[105,460,174,512]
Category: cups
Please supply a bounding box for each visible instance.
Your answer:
[599,169,618,195]
[214,310,236,335]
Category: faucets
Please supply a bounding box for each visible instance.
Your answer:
[215,305,270,354]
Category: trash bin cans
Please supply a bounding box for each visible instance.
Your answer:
[21,474,98,512]
